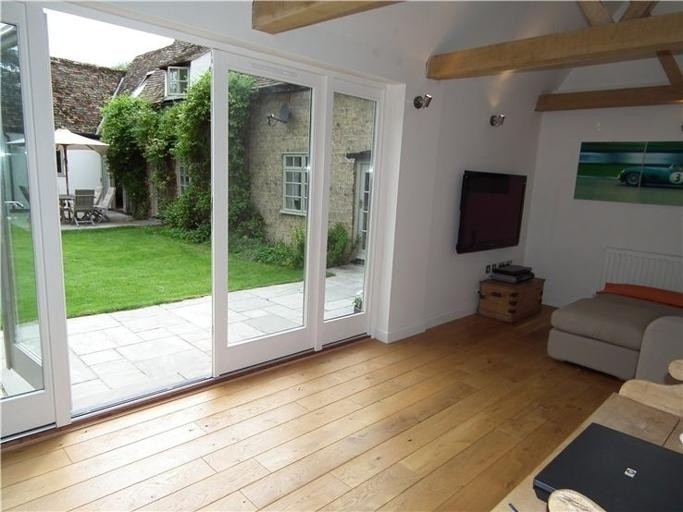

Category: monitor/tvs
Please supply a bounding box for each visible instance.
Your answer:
[455,170,527,254]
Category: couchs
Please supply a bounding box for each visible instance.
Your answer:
[544,247,682,382]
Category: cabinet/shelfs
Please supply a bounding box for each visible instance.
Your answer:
[476,277,545,324]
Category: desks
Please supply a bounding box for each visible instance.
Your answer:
[487,359,683,512]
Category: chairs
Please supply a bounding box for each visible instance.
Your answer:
[17,186,117,227]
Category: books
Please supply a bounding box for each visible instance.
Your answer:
[491,264,535,285]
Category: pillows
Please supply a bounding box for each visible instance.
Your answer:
[653,288,682,308]
[599,279,658,302]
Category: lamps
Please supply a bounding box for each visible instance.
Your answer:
[265,112,291,127]
[489,113,505,127]
[412,94,432,110]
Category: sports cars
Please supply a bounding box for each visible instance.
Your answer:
[614,160,682,192]
